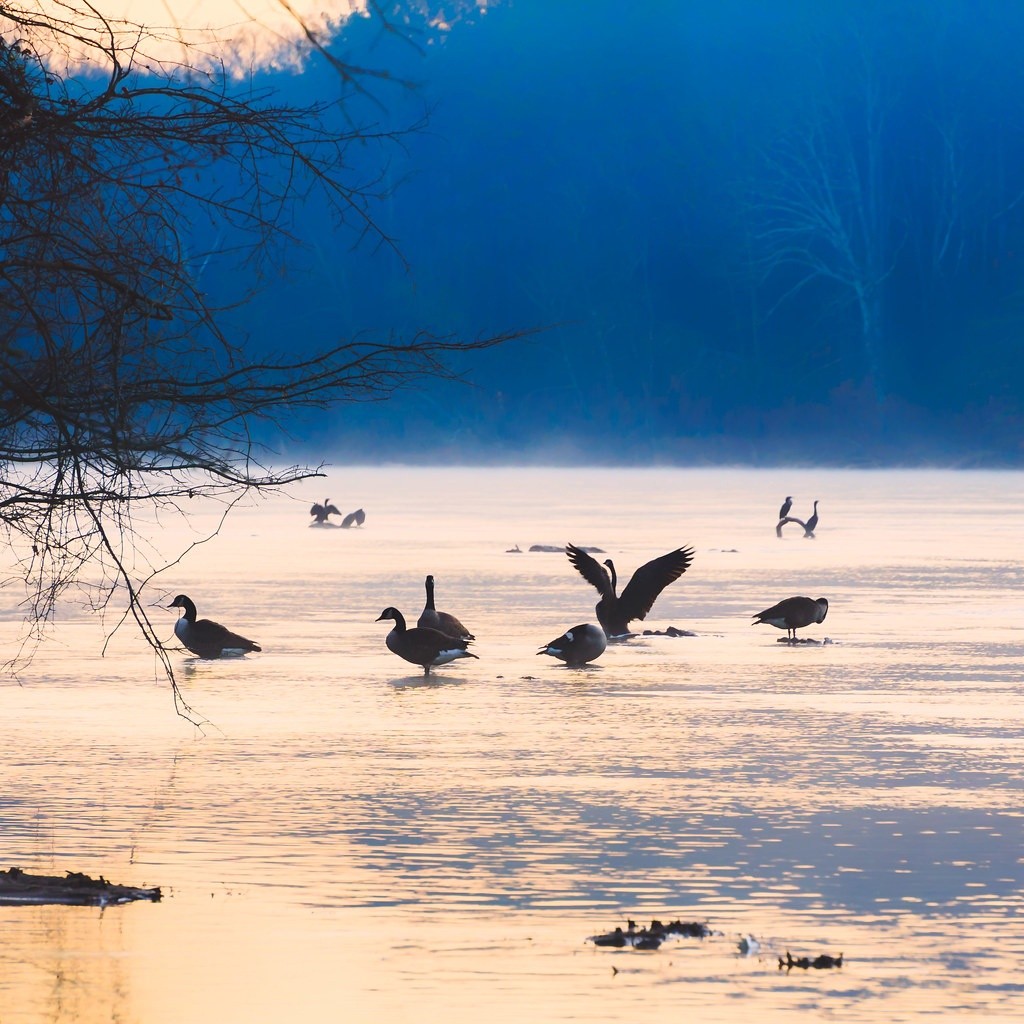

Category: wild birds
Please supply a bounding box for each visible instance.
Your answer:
[803,500,819,537]
[166,594,262,660]
[750,595,828,641]
[536,542,697,664]
[779,497,793,519]
[375,574,480,680]
[310,498,365,529]
